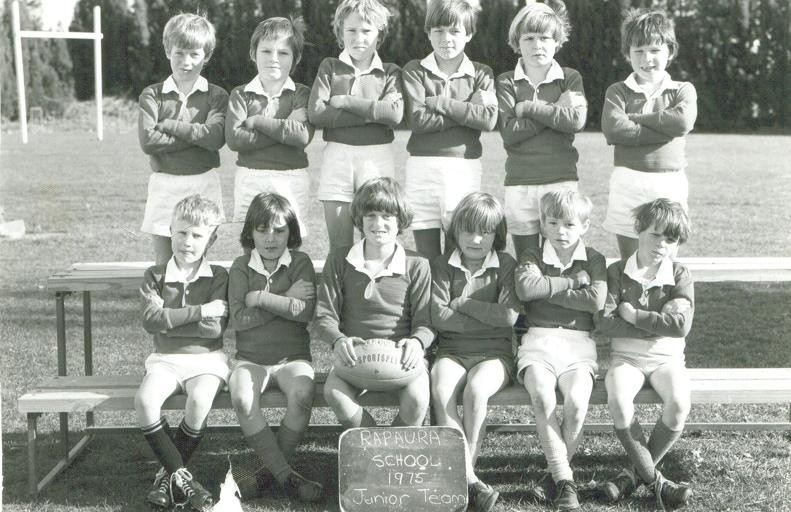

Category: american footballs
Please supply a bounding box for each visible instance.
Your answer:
[332,337,420,390]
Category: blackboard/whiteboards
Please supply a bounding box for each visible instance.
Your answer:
[337,425,470,512]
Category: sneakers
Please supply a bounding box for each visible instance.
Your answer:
[555,479,580,511]
[532,472,557,504]
[235,466,274,503]
[603,465,642,503]
[149,467,173,509]
[280,471,323,505]
[468,481,499,512]
[170,468,213,512]
[644,468,691,512]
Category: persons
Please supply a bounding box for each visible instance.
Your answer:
[401,1,499,260]
[496,1,588,268]
[513,186,608,509]
[133,194,229,512]
[427,191,521,511]
[225,192,324,502]
[137,11,229,265]
[310,176,439,428]
[601,9,697,259]
[307,0,406,262]
[225,17,315,263]
[594,197,695,506]
